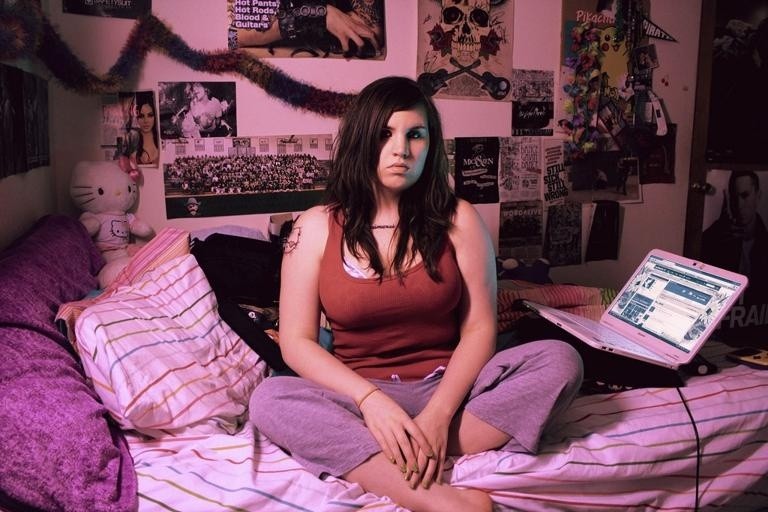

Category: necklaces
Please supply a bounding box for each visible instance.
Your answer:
[371,225,396,230]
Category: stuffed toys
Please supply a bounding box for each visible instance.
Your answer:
[68,155,156,289]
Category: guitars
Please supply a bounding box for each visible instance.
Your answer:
[448,57,510,100]
[417,59,481,96]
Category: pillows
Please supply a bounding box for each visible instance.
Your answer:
[0,212,139,512]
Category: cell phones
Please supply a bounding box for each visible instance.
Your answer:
[726,346,768,369]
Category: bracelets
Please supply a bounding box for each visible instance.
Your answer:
[357,387,381,408]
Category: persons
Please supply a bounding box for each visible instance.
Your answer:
[184,197,201,219]
[190,83,223,137]
[167,153,321,194]
[124,101,158,164]
[247,75,585,512]
[698,169,768,310]
[236,0,384,58]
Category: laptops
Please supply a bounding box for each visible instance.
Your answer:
[522,248,750,373]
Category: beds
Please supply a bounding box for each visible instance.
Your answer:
[0,278,767,511]
